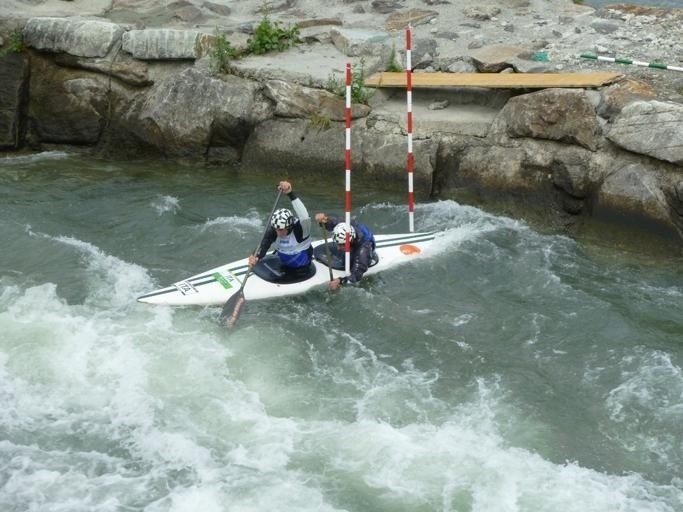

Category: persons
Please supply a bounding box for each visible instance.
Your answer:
[316,213,375,290]
[250,181,314,268]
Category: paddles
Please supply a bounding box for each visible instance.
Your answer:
[218,184,283,333]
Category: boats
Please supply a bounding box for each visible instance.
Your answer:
[136,229,459,306]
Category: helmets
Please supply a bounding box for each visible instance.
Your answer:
[271,208,299,230]
[333,222,356,244]
